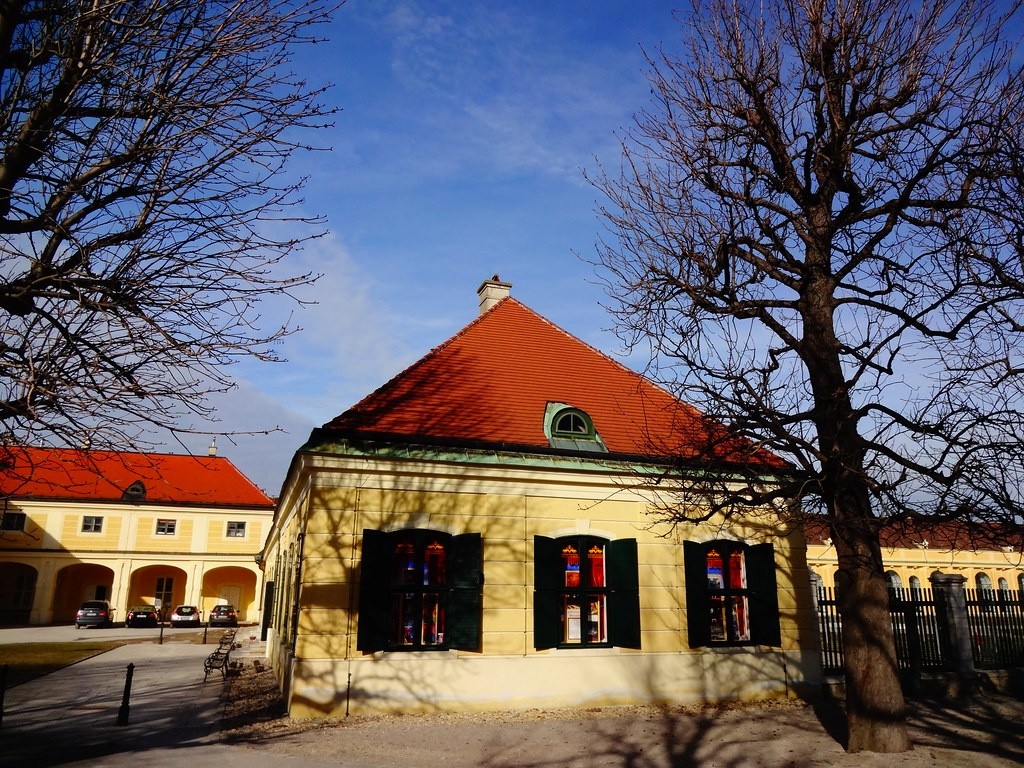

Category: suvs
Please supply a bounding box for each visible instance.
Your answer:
[71,599,117,630]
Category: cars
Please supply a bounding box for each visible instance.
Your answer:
[169,605,203,628]
[125,604,162,628]
[208,604,241,628]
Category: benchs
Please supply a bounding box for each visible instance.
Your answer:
[219,627,239,650]
[204,644,234,682]
